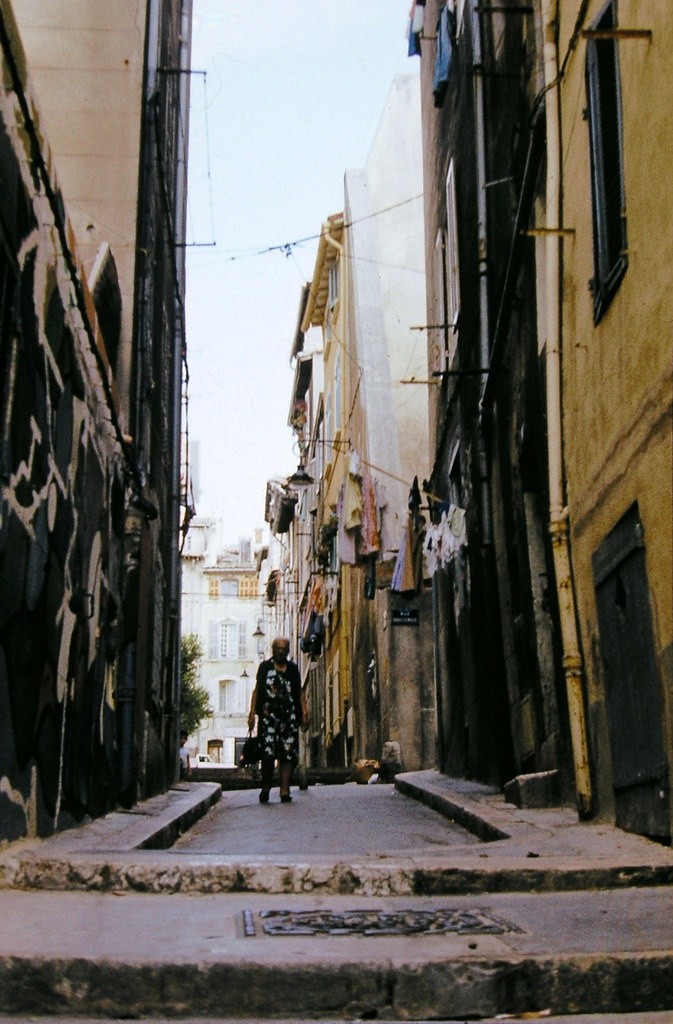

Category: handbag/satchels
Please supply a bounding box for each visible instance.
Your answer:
[243,728,262,763]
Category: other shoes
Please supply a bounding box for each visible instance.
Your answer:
[280,793,292,803]
[259,790,268,803]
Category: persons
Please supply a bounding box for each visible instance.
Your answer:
[177,730,193,782]
[246,638,310,804]
